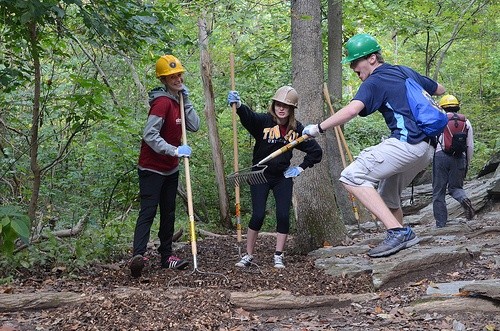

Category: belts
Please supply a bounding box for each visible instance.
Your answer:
[422,136,437,149]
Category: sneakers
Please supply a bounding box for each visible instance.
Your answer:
[366,225,420,257]
[274,252,285,269]
[462,198,476,221]
[130,254,145,279]
[162,255,189,270]
[235,252,253,268]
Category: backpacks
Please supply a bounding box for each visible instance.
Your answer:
[382,64,449,139]
[436,114,469,156]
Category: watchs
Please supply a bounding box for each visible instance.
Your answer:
[318,122,327,134]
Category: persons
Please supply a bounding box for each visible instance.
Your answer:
[227,86,323,269]
[302,32,446,257]
[130,54,200,277]
[431,94,476,227]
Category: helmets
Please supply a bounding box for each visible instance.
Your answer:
[342,33,382,64]
[439,95,459,108]
[155,55,187,78]
[270,86,299,108]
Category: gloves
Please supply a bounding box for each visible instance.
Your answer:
[283,166,305,179]
[175,145,192,157]
[227,91,242,109]
[302,124,327,142]
[178,84,189,99]
